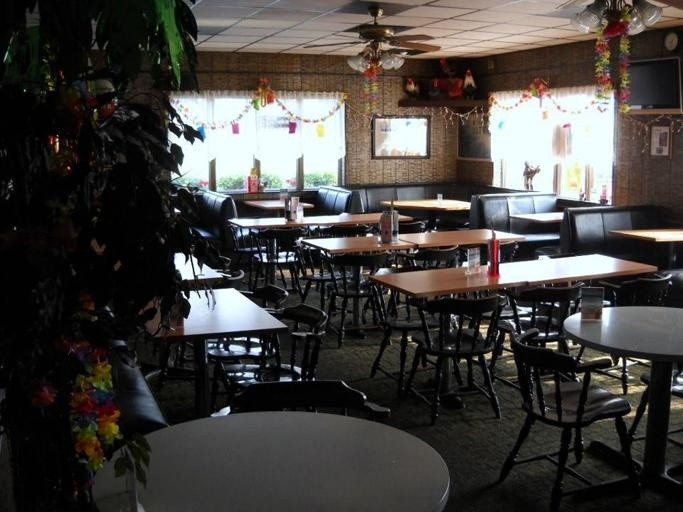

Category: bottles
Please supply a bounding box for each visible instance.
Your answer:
[488,232,500,276]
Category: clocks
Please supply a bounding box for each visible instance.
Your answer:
[662,33,680,52]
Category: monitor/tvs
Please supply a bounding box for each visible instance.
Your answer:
[625,56,683,109]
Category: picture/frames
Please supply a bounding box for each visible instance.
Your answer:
[371,113,431,159]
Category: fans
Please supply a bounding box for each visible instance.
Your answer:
[557,0,683,14]
[302,6,442,55]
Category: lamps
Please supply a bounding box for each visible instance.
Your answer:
[346,42,405,75]
[574,0,663,42]
